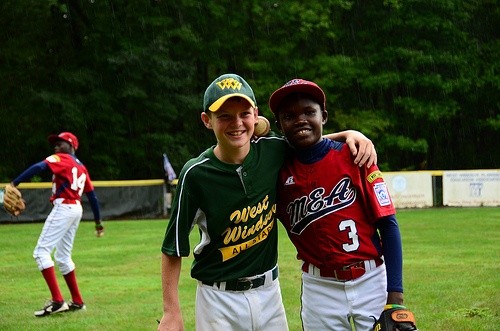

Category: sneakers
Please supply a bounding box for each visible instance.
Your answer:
[68,301,85,312]
[34,300,70,317]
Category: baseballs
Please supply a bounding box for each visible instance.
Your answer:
[95,231,104,237]
[253,116,270,135]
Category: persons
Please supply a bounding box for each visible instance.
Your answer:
[157,74,377,331]
[0,132,103,317]
[268,78,417,331]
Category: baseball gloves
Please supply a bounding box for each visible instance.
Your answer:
[371,304,417,331]
[0,184,26,217]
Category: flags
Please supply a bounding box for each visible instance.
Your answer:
[163,154,176,181]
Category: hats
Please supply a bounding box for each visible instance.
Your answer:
[269,79,325,116]
[48,132,79,150]
[203,73,257,112]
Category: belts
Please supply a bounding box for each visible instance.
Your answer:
[301,256,384,281]
[62,199,76,204]
[202,267,279,291]
